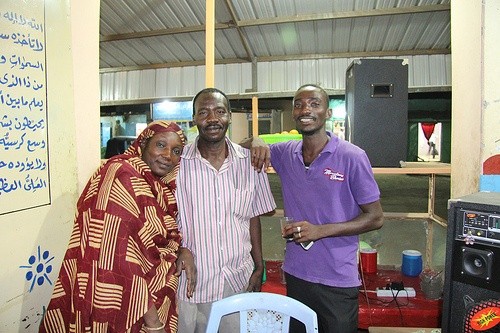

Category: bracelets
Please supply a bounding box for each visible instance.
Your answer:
[143,321,166,331]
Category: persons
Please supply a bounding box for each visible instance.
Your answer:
[38,119,198,333]
[248,84,385,333]
[334,121,347,140]
[427,140,437,160]
[174,87,278,333]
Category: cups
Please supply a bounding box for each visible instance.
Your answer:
[280,217,294,241]
[359,248,377,274]
[254,260,266,284]
[401,250,423,276]
[278,263,287,284]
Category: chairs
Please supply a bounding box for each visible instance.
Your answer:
[206,292,318,332]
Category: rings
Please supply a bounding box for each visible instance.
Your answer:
[297,226,302,233]
[298,232,302,239]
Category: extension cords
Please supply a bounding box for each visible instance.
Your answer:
[375,285,416,298]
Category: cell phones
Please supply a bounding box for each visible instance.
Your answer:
[294,233,314,251]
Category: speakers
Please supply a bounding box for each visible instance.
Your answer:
[441,190,500,333]
[343,57,410,168]
